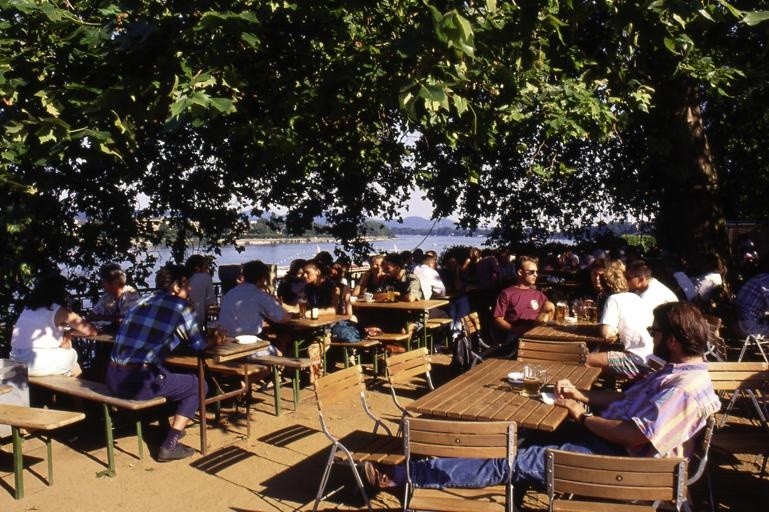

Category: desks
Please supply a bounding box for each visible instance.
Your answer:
[408,356,606,436]
[523,311,606,341]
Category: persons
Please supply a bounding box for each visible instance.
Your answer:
[438,232,769,383]
[9,248,449,461]
[340,302,723,488]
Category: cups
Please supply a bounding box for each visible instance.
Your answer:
[554,298,598,326]
[385,286,395,302]
[363,292,373,302]
[298,295,308,320]
[207,321,228,346]
[522,364,546,398]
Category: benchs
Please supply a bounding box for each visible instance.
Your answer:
[368,333,410,358]
[248,353,313,418]
[29,375,167,477]
[330,339,380,370]
[0,404,86,499]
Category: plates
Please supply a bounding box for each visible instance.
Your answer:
[88,321,113,327]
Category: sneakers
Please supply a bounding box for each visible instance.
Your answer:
[154,442,195,462]
[361,461,405,498]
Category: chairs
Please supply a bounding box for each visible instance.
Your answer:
[514,338,590,367]
[314,363,406,511]
[459,312,514,371]
[544,447,690,511]
[384,347,435,437]
[698,361,768,479]
[408,416,519,512]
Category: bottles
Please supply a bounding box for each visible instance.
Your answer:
[311,294,319,320]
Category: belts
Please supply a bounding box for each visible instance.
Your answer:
[111,361,143,369]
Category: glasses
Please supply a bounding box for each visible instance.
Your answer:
[647,327,666,337]
[623,271,641,281]
[523,270,539,275]
